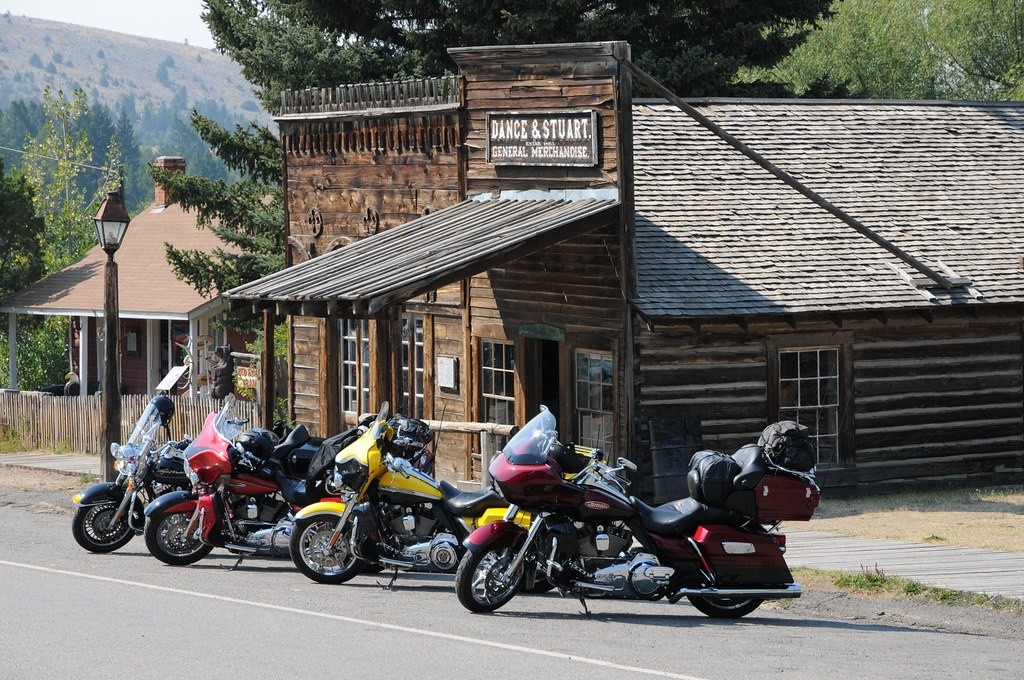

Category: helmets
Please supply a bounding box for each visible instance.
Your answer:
[150,397,175,426]
[549,443,590,473]
[238,427,273,460]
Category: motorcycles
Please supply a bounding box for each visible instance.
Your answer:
[72,396,293,554]
[289,401,608,595]
[145,394,433,570]
[457,406,821,617]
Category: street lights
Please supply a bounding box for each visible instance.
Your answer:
[90,191,131,479]
